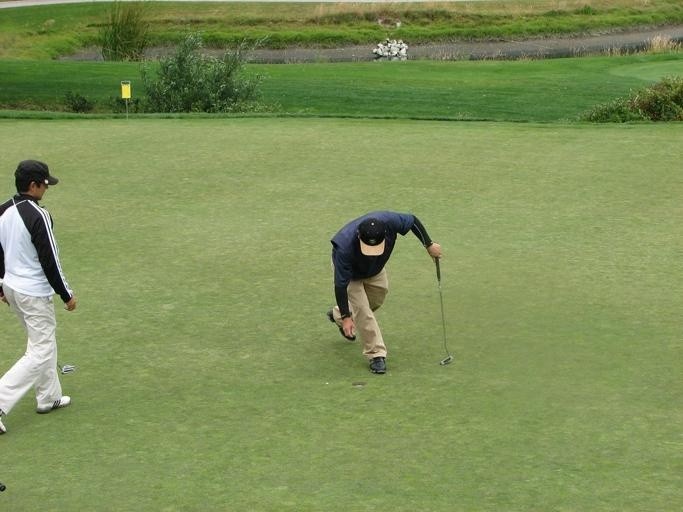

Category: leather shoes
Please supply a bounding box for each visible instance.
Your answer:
[327,310,356,341]
[369,356,386,374]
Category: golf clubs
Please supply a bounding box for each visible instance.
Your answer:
[4,299,75,375]
[435,241,454,366]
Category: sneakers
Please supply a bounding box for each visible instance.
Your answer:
[36,395,70,414]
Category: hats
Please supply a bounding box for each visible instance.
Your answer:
[358,218,386,256]
[14,159,59,185]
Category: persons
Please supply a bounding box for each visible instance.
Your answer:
[0,158,78,436]
[324,208,441,375]
